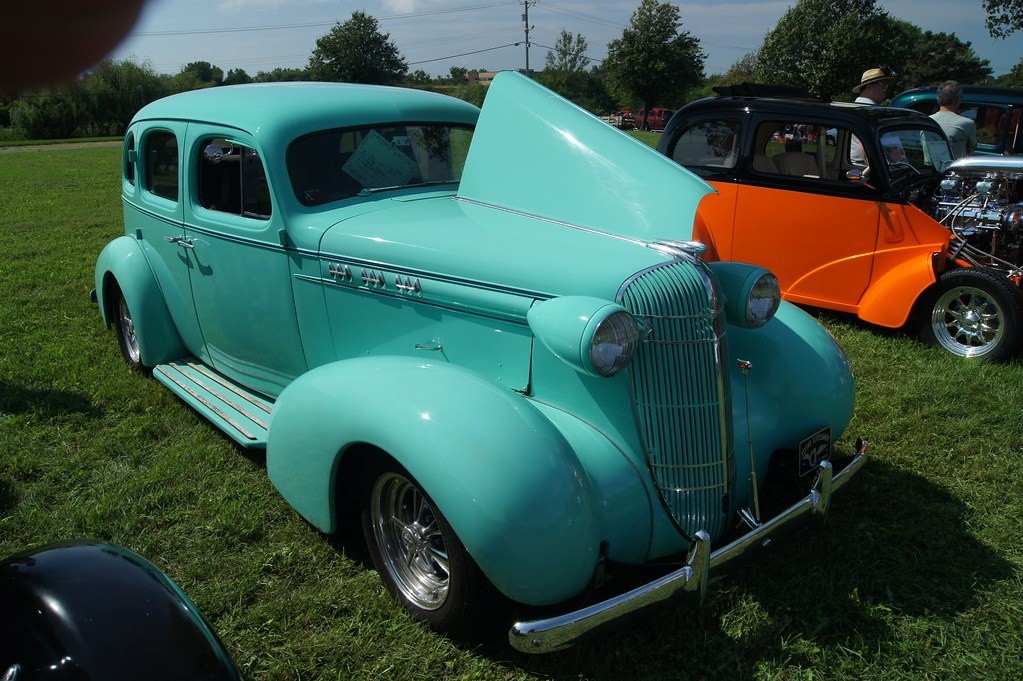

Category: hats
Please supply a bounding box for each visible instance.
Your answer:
[852,69,895,93]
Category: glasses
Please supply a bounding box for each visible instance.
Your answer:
[874,82,887,87]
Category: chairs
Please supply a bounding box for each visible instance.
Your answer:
[772,152,819,176]
[723,151,780,173]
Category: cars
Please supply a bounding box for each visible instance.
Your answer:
[615,110,638,130]
[94,68,870,653]
[889,86,1023,166]
[654,81,1023,358]
[1,538,242,680]
[635,107,674,130]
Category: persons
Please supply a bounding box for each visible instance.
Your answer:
[849,67,904,166]
[920,81,977,174]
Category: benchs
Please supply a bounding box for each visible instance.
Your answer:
[203,155,257,210]
